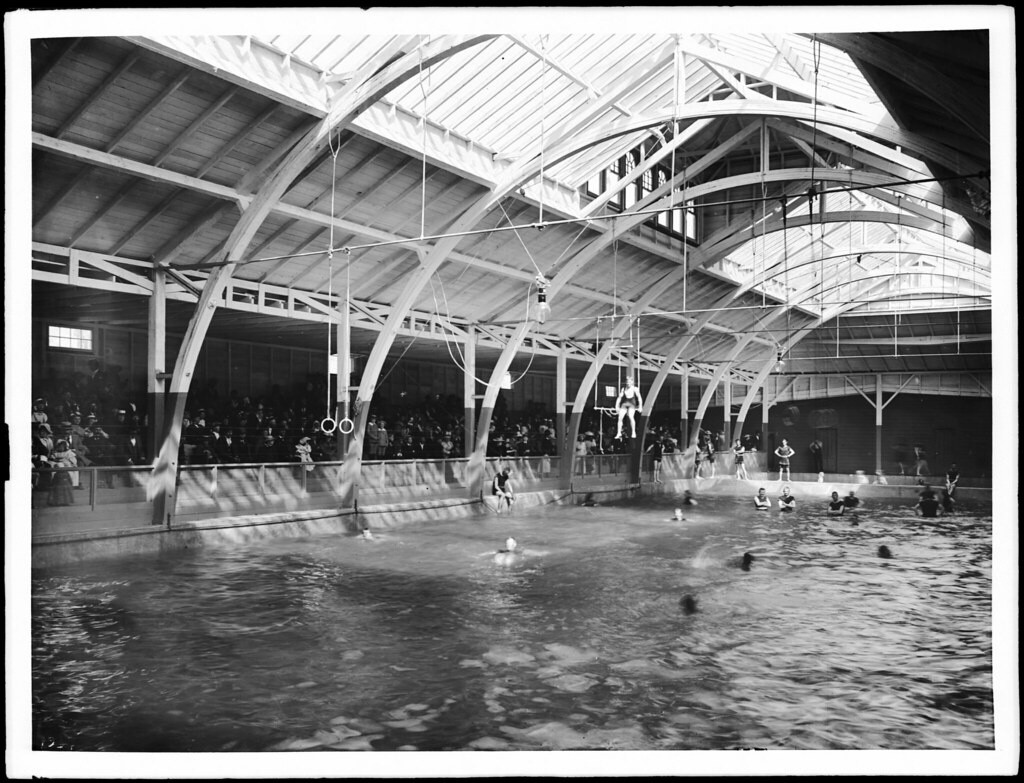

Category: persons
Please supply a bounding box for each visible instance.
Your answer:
[683,488,692,502]
[645,432,665,482]
[615,375,643,439]
[753,486,772,511]
[695,438,705,479]
[913,484,944,517]
[672,509,685,521]
[734,438,751,481]
[827,489,846,516]
[842,491,865,506]
[811,436,824,471]
[505,537,517,552]
[706,438,716,478]
[778,488,797,514]
[893,441,933,476]
[492,468,517,513]
[29,370,772,504]
[942,472,957,509]
[756,430,762,451]
[774,437,795,482]
